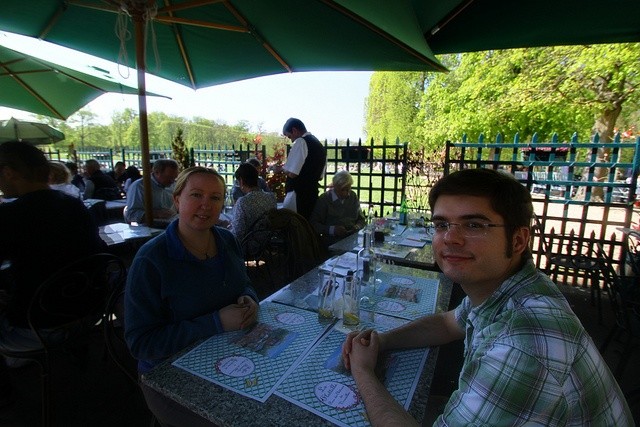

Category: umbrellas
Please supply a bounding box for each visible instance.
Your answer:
[0,45,171,120]
[417,0,640,55]
[0,2,452,225]
[1,117,65,145]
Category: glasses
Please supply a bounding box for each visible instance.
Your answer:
[426,218,506,239]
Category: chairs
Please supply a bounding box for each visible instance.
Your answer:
[625,230,640,274]
[1,252,126,377]
[93,188,122,201]
[101,272,163,426]
[242,213,276,293]
[598,242,640,383]
[533,211,607,326]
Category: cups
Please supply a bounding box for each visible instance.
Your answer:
[317,271,336,320]
[342,275,361,327]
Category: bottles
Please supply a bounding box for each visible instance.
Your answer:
[356,231,376,309]
[399,194,408,225]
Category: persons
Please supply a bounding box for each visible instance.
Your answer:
[110,161,126,184]
[123,166,259,367]
[282,118,327,216]
[343,168,634,426]
[47,162,83,199]
[232,158,265,202]
[65,162,82,186]
[317,171,366,237]
[1,140,112,349]
[81,156,115,201]
[230,163,277,238]
[123,159,180,224]
